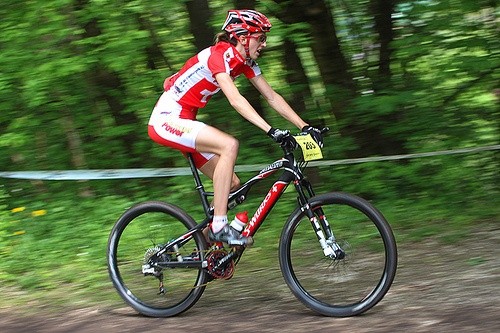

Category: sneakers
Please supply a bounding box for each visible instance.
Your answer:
[209,222,254,245]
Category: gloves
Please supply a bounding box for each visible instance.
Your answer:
[302,126,324,148]
[267,127,298,155]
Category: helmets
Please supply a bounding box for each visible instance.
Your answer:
[222,10,272,36]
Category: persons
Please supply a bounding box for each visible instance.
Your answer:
[148,10,323,251]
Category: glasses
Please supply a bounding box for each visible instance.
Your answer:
[245,34,267,43]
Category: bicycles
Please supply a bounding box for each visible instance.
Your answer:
[106,127,398,318]
